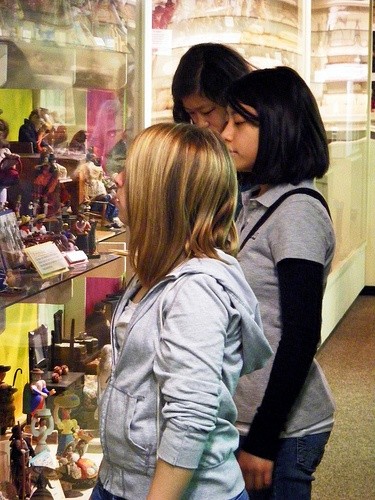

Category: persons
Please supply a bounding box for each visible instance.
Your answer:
[88,123,273,500]
[171,43,260,137]
[219,67,334,500]
[0,96,120,497]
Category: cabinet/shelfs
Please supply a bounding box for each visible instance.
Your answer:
[0,0,375,500]
